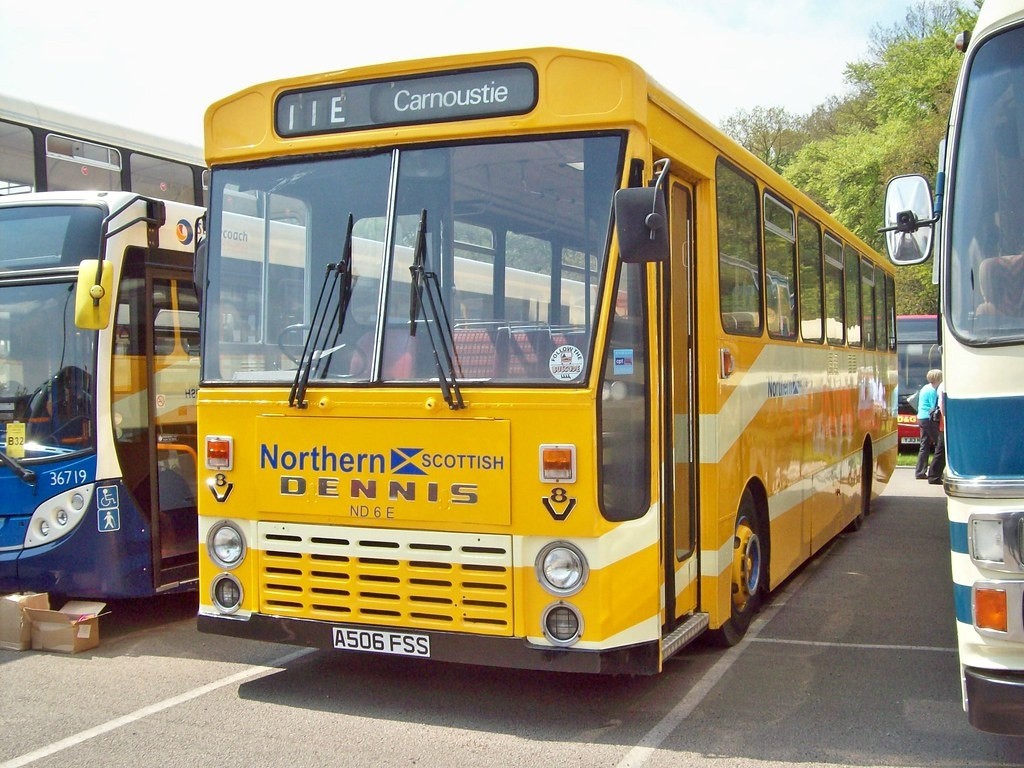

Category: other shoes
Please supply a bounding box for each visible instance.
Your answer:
[916,476,928,479]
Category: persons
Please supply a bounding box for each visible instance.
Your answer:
[927,380,946,485]
[914,368,943,480]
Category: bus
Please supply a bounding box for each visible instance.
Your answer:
[719,251,792,337]
[881,0,1024,736]
[194,46,902,680]
[854,314,948,447]
[1,190,640,600]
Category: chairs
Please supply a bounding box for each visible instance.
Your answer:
[975,255,1024,321]
[721,310,792,337]
[353,319,648,433]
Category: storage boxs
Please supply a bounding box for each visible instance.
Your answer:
[24,600,112,654]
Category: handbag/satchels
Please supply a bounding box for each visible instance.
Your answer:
[907,391,919,412]
[929,397,941,424]
